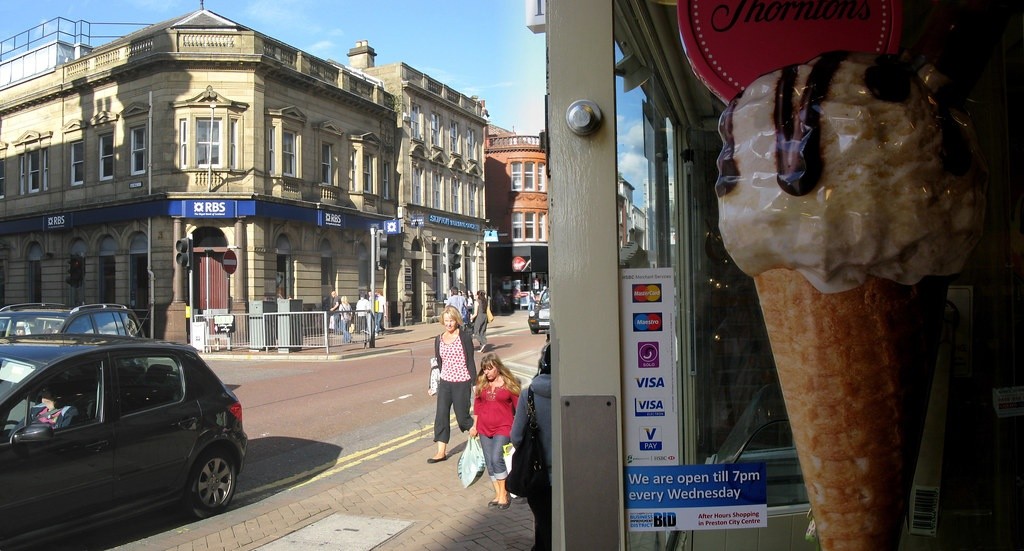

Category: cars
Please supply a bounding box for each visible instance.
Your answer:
[514,291,530,309]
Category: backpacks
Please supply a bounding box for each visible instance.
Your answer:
[340,304,352,324]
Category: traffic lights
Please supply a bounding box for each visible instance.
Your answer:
[447,242,461,267]
[375,230,391,269]
[176,238,192,268]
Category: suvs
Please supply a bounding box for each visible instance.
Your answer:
[0,302,147,370]
[0,334,248,551]
[528,288,549,334]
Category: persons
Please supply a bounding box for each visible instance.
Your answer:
[445,286,488,353]
[7,376,81,439]
[340,295,353,344]
[427,305,478,464]
[510,344,553,551]
[355,289,386,336]
[469,353,522,510]
[329,290,341,335]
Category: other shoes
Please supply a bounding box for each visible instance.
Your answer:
[488,500,499,507]
[477,345,488,353]
[498,498,512,510]
[427,454,447,463]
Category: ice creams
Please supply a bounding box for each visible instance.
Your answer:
[716,50,991,551]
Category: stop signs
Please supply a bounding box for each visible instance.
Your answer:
[222,250,237,274]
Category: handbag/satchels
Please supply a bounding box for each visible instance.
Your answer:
[485,305,494,323]
[503,386,552,498]
[457,435,486,489]
[348,322,355,334]
[427,357,441,397]
[328,315,334,329]
[502,442,519,498]
